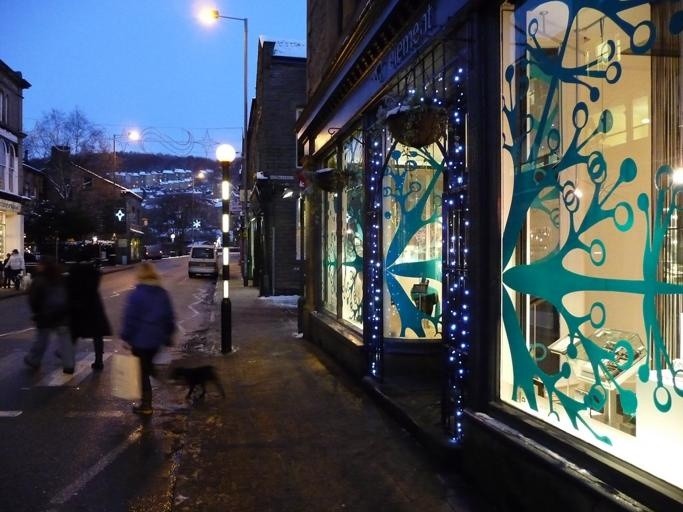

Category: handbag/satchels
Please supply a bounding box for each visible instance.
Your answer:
[110,343,142,400]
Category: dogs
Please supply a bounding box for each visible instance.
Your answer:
[167,364,227,404]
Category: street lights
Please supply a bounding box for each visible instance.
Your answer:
[190,170,206,240]
[195,3,251,285]
[112,130,141,241]
[214,141,236,356]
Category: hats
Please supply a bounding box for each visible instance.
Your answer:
[135,263,160,280]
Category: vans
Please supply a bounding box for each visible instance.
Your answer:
[187,243,218,279]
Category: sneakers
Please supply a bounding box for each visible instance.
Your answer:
[132,403,153,415]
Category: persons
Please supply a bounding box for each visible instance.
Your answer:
[4,254,11,288]
[5,249,25,291]
[120,262,177,415]
[53,260,113,370]
[23,257,75,374]
